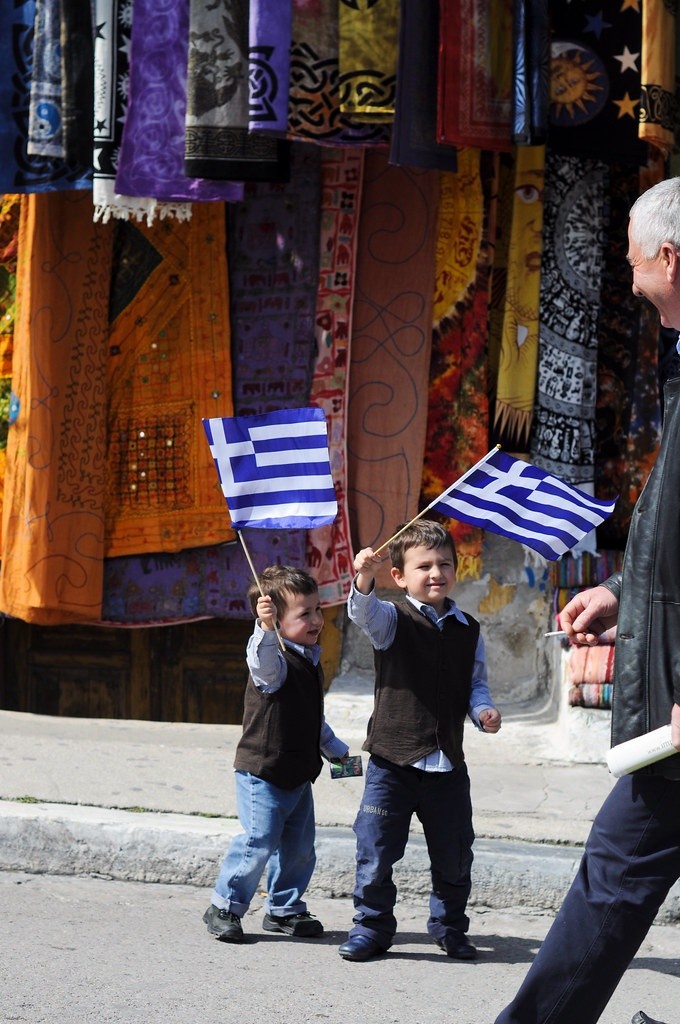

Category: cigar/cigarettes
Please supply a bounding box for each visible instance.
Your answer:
[544,631,566,638]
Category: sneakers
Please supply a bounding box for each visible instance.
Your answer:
[262,911,323,937]
[202,904,243,942]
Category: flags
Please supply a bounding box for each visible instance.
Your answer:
[201,407,338,531]
[429,450,620,563]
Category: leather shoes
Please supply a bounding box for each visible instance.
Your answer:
[338,935,387,961]
[631,1010,665,1024]
[434,932,478,959]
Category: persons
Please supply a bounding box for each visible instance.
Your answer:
[492,174,680,1023]
[337,521,501,963]
[203,561,350,943]
